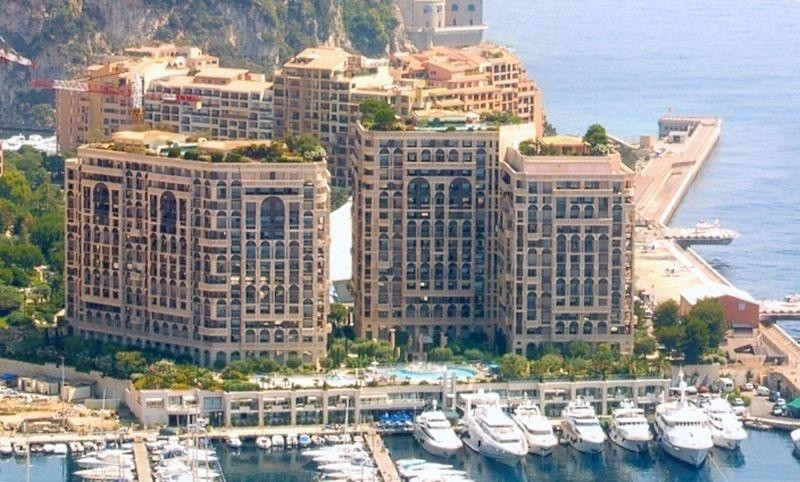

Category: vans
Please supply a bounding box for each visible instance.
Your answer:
[745,383,754,390]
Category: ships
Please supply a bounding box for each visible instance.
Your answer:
[413,399,463,456]
[559,395,608,454]
[456,393,558,467]
[654,365,747,468]
[609,398,654,452]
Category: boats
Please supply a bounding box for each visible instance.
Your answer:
[662,227,739,242]
[255,432,384,482]
[395,458,473,482]
[745,421,773,430]
[754,293,800,318]
[226,435,241,448]
[0,440,136,482]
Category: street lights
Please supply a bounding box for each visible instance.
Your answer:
[55,356,66,428]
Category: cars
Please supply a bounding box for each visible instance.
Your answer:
[757,387,787,416]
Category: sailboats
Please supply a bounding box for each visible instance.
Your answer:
[144,401,222,482]
